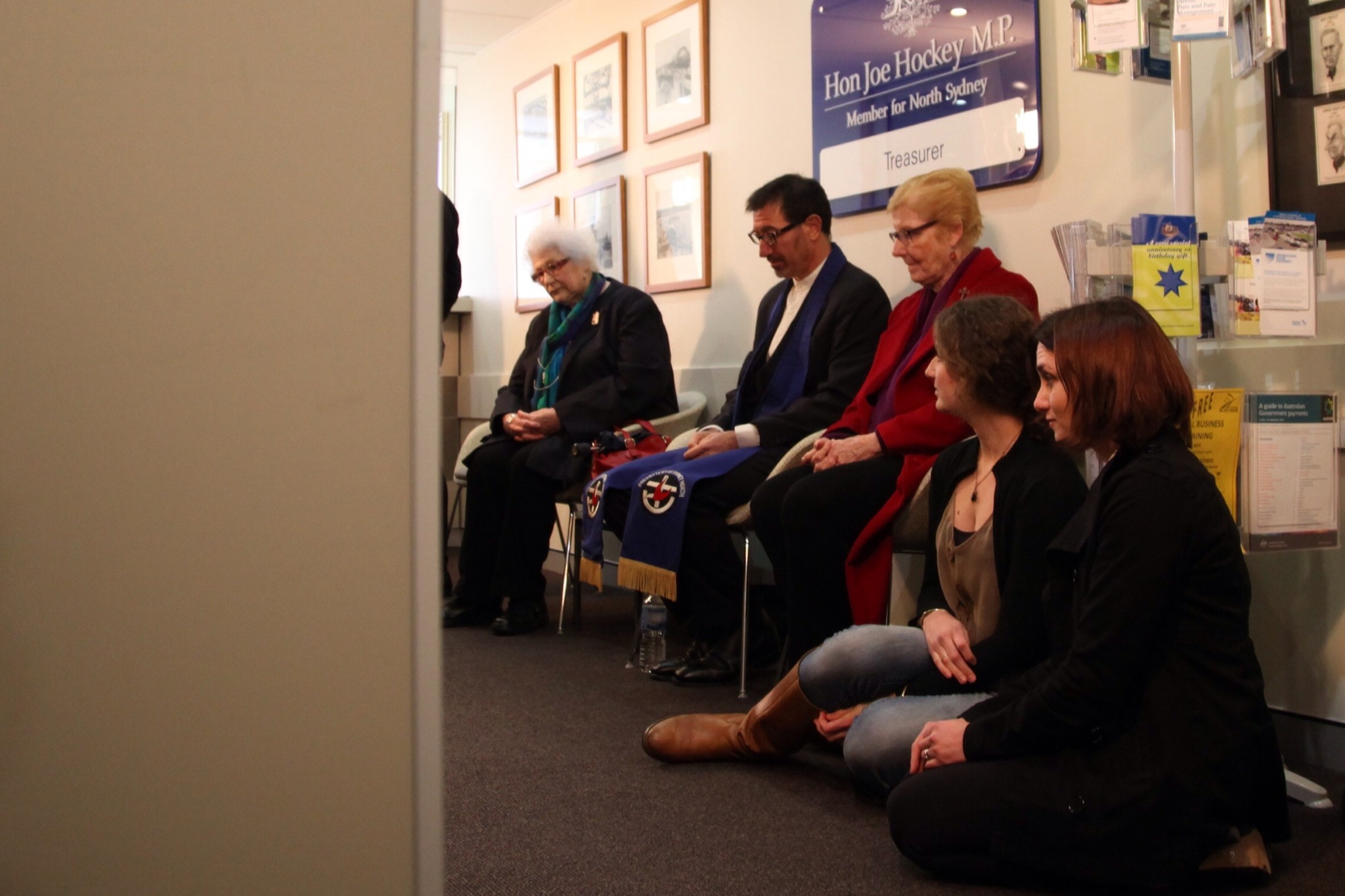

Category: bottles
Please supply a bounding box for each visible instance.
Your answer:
[637,593,666,671]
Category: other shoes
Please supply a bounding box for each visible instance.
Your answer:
[441,599,503,628]
[491,600,550,636]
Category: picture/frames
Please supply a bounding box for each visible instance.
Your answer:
[513,196,560,312]
[643,150,711,293]
[511,64,561,189]
[569,175,628,286]
[572,32,627,168]
[641,0,710,145]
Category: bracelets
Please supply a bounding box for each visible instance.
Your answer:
[918,607,949,628]
[506,413,518,423]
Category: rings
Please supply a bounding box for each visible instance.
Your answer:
[941,653,947,662]
[924,748,930,760]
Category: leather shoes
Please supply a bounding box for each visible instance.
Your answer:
[648,646,704,681]
[673,631,744,686]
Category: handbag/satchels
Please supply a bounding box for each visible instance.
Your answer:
[591,419,673,480]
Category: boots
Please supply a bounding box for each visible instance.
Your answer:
[641,649,831,763]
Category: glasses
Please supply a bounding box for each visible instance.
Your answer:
[889,219,940,245]
[531,257,572,283]
[747,215,808,245]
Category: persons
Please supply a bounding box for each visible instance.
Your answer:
[442,193,463,320]
[642,297,1086,792]
[647,173,893,682]
[751,167,1041,661]
[442,220,679,636]
[888,298,1291,896]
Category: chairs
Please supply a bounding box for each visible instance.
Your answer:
[444,390,1044,701]
